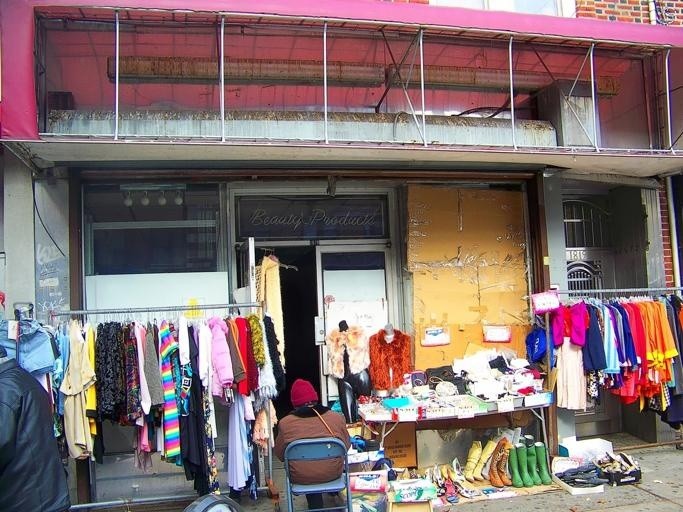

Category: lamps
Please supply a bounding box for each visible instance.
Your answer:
[120,183,187,207]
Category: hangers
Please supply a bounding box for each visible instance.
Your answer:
[566,287,681,306]
[49,302,264,325]
[262,248,299,272]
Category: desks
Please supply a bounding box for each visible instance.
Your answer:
[358,402,551,478]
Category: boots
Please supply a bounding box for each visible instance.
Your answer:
[527,443,542,485]
[482,454,493,480]
[535,442,552,485]
[516,444,533,487]
[474,440,497,481]
[490,437,508,488]
[498,443,512,487]
[463,441,482,482]
[510,445,523,487]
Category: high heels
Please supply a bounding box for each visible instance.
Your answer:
[433,463,445,487]
[454,479,471,498]
[453,458,465,481]
[447,463,460,482]
[444,480,458,502]
[440,464,449,481]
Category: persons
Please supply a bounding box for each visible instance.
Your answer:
[0,342,70,512]
[325,320,370,425]
[276,378,348,512]
[368,324,415,398]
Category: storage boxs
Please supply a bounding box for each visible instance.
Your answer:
[374,424,418,469]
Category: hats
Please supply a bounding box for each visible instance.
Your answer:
[290,379,319,406]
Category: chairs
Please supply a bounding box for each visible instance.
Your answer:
[280,437,351,511]
